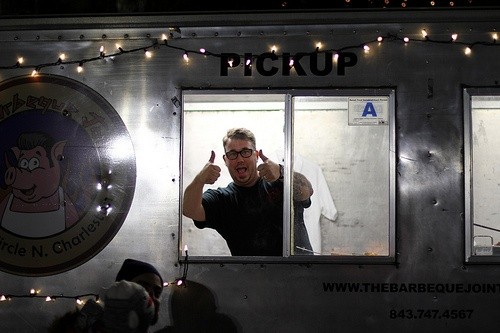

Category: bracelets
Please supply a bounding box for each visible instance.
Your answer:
[278,163,284,179]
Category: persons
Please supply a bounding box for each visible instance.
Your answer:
[47,259,164,333]
[182,128,313,256]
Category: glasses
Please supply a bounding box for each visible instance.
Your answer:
[226,148,254,160]
